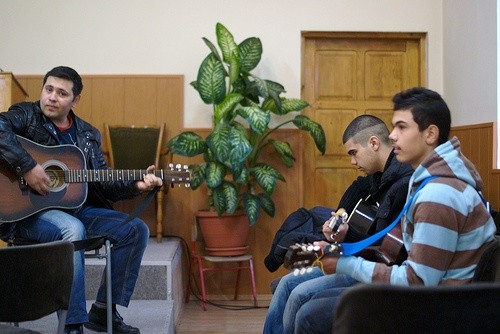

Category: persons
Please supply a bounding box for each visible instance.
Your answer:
[0,66,163,334]
[283,86,496,334]
[263,115,415,334]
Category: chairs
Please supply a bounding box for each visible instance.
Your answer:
[104,121,165,243]
[186,208,258,311]
[7,235,112,334]
[0,239,74,334]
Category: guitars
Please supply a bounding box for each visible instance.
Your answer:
[0,133,191,227]
[327,193,380,243]
[281,220,409,277]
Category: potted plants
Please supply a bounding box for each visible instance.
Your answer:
[167,22,327,256]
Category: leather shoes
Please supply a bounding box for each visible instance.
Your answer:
[63,324,84,334]
[84,304,141,334]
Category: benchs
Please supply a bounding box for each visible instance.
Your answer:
[332,235,500,334]
